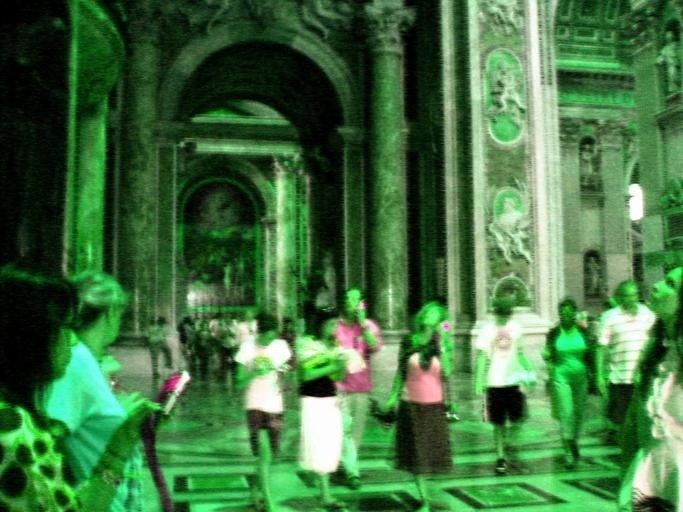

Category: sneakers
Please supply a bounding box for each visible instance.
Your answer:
[495,459,530,475]
[565,452,592,471]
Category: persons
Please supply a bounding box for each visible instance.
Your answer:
[581,144,598,182]
[315,253,336,309]
[228,288,461,511]
[499,65,525,112]
[473,285,598,475]
[597,267,682,512]
[490,198,532,265]
[178,310,248,379]
[1,269,193,511]
[660,31,679,92]
[587,257,604,295]
